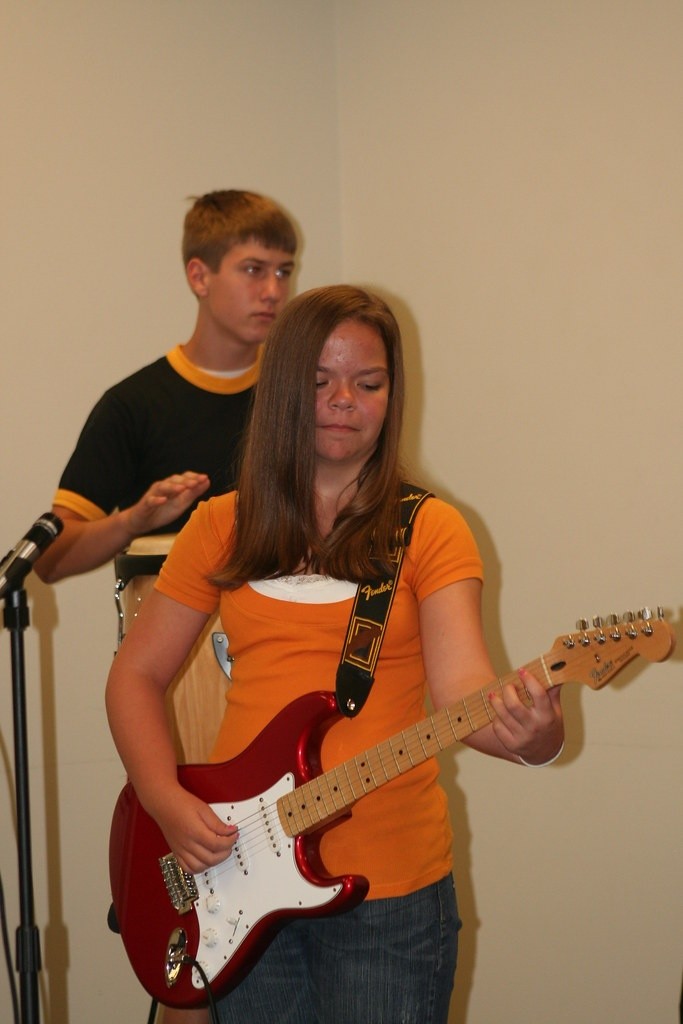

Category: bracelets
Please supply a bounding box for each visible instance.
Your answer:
[518,741,564,767]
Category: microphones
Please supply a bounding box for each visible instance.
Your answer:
[0,511,65,599]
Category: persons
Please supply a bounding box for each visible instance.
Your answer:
[34,189,298,1024]
[105,284,565,1024]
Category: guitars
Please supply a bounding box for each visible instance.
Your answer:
[107,605,676,1010]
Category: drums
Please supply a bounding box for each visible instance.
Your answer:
[114,534,233,769]
